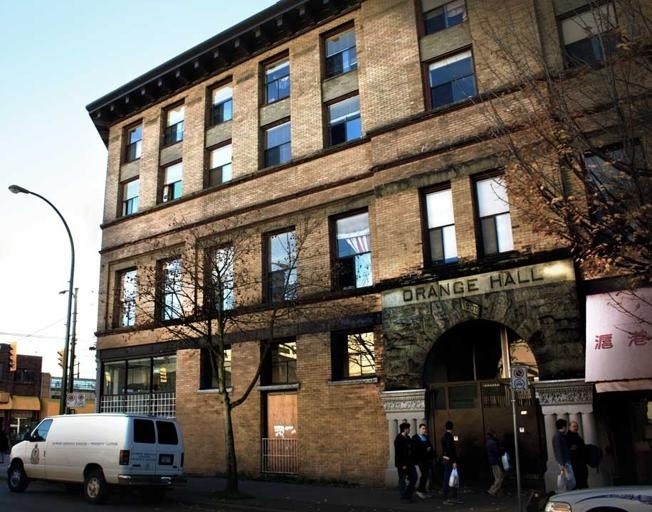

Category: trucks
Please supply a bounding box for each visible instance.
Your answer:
[6,412,187,502]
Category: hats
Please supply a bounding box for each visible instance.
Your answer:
[486,424,497,433]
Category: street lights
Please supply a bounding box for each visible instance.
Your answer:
[57,285,79,415]
[7,184,76,412]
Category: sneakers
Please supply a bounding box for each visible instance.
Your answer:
[400,498,418,504]
[415,490,433,500]
[449,498,465,504]
[440,499,456,507]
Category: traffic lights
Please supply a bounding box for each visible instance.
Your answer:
[6,342,16,372]
[58,350,64,367]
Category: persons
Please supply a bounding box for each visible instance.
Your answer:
[567,420,589,491]
[393,422,419,505]
[439,420,465,507]
[0,426,10,464]
[411,423,435,500]
[551,418,577,491]
[485,426,507,499]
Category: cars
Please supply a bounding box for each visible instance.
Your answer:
[541,484,652,511]
